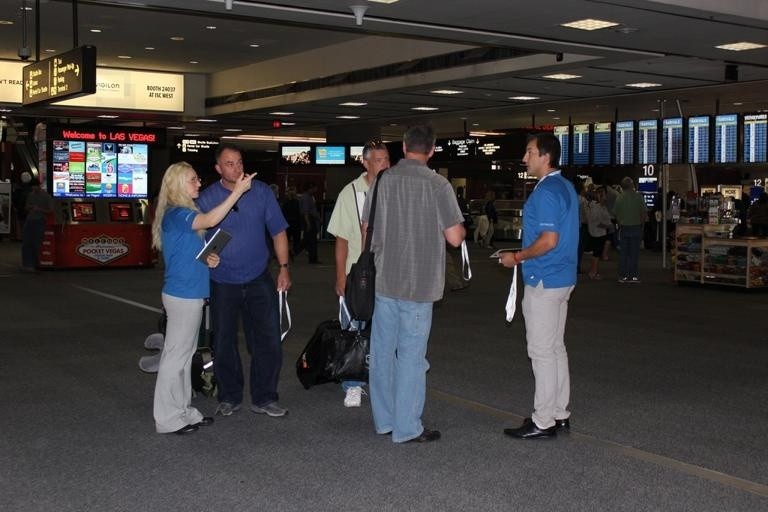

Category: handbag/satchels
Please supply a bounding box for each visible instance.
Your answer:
[580,200,590,224]
[295,320,371,390]
[345,252,374,321]
[292,240,305,256]
[159,301,217,336]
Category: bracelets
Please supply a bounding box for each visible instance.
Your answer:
[513,252,519,263]
[279,264,290,269]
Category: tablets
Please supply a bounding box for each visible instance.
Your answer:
[489,248,526,263]
[196,228,232,266]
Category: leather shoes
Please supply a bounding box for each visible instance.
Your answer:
[409,431,440,443]
[503,417,570,440]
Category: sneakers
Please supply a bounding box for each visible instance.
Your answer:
[173,417,213,435]
[214,401,242,416]
[250,400,288,417]
[343,386,361,408]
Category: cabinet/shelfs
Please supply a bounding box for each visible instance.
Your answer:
[700,239,768,288]
[674,224,737,283]
[469,199,525,240]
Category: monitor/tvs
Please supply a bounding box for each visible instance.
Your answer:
[348,144,365,166]
[717,185,744,202]
[700,185,717,198]
[47,139,152,199]
[279,143,314,168]
[314,143,348,167]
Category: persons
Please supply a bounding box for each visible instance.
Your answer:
[299,181,324,263]
[734,191,768,238]
[327,138,390,407]
[496,132,579,439]
[479,191,498,249]
[150,160,259,435]
[361,124,466,444]
[21,177,55,271]
[194,142,293,418]
[282,188,302,263]
[577,176,647,284]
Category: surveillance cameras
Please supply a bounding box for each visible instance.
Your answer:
[18,47,32,61]
